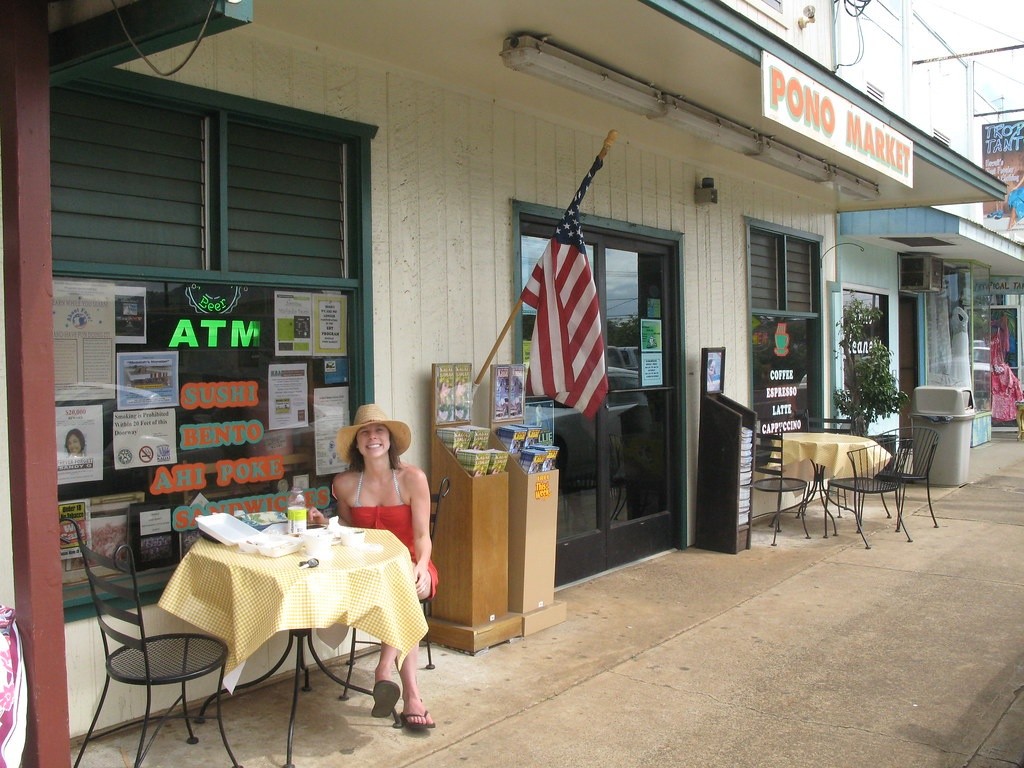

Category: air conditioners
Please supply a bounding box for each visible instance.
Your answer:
[898,253,945,295]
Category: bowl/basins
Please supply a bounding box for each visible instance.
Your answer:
[340,529,365,547]
[195,511,303,558]
[304,530,332,554]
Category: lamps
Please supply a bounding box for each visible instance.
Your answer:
[797,4,817,31]
[498,33,666,116]
[818,163,880,202]
[647,92,759,155]
[747,136,829,185]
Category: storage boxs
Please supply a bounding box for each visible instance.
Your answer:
[195,512,306,556]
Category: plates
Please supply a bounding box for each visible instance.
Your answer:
[237,511,288,529]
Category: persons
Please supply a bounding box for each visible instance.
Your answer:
[65,428,86,458]
[307,404,439,728]
[950,307,971,407]
[536,404,544,427]
[437,376,470,422]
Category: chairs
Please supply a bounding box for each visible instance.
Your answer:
[59,518,242,768]
[752,428,811,549]
[332,478,452,701]
[798,409,854,518]
[860,425,941,532]
[822,434,916,549]
[609,433,653,520]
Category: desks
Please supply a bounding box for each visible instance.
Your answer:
[776,432,892,536]
[157,524,429,768]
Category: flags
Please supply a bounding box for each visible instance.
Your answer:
[520,156,609,419]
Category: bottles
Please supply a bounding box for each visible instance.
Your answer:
[287,487,306,534]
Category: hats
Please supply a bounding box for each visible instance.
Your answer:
[335,404,411,463]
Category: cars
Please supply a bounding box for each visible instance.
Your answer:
[546,365,639,490]
[58,379,168,494]
[973,340,991,391]
[605,345,639,368]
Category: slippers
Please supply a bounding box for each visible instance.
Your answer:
[401,711,435,729]
[371,680,400,717]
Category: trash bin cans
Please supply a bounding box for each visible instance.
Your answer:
[907,385,977,486]
